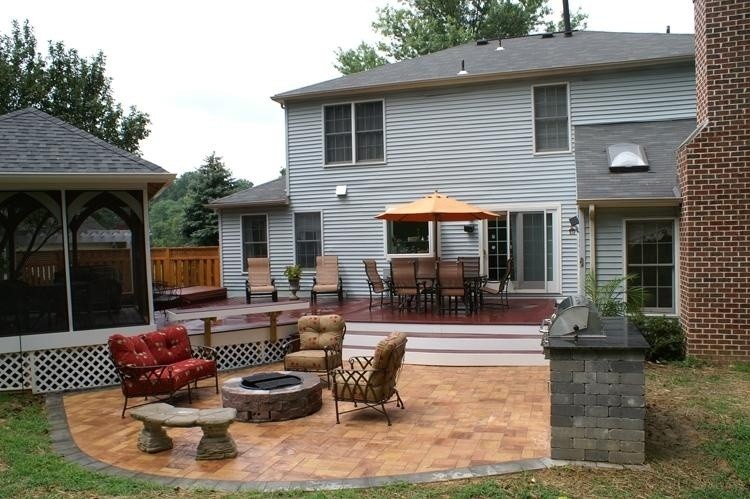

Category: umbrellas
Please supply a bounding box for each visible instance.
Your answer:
[372,189,501,261]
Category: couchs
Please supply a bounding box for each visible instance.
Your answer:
[108,326,218,418]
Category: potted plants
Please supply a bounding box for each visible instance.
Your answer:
[283,264,303,300]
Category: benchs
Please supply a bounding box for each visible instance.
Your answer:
[130,402,238,461]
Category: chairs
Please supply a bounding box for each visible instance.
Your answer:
[329,331,408,426]
[311,256,343,305]
[245,258,277,304]
[363,256,512,312]
[282,314,346,390]
